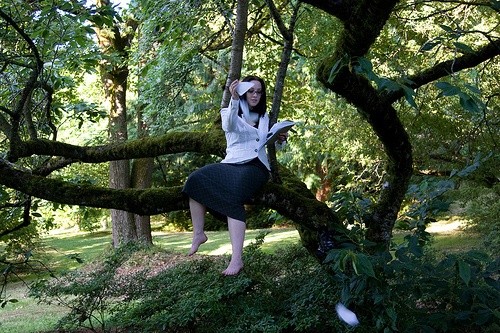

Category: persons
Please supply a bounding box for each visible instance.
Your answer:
[181,75,289,276]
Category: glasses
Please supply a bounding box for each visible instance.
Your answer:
[247,88,263,94]
